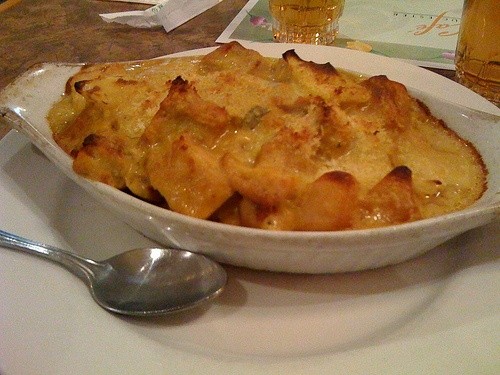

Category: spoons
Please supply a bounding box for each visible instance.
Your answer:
[1,229,227,316]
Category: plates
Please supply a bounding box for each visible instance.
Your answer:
[1,43,499,274]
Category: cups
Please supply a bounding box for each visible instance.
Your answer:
[455,0,500,108]
[268,0,344,46]
